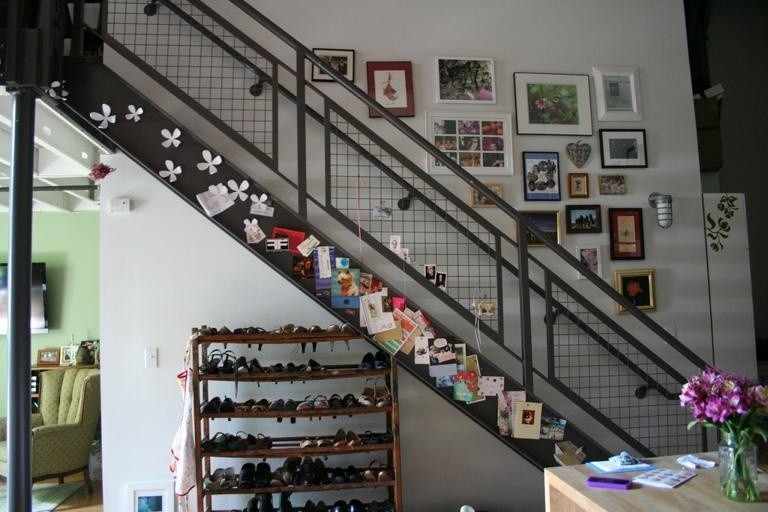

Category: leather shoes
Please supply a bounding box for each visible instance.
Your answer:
[314,456,332,486]
[316,437,331,448]
[283,455,302,487]
[345,465,363,483]
[317,499,328,512]
[239,462,256,489]
[303,498,317,512]
[270,464,287,487]
[331,500,347,512]
[235,399,256,413]
[332,466,347,485]
[307,359,321,375]
[360,352,376,371]
[382,429,392,442]
[348,500,363,512]
[254,462,272,488]
[359,428,380,446]
[374,350,390,370]
[298,438,314,448]
[251,398,270,413]
[293,454,314,485]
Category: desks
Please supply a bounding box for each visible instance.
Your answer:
[542,450,768,512]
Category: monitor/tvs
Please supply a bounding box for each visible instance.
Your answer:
[0,262,49,335]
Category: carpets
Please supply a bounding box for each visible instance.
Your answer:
[0,482,88,512]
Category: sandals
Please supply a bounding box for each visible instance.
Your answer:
[283,323,296,337]
[272,325,283,336]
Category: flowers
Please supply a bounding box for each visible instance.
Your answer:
[678,370,768,501]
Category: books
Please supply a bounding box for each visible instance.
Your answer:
[633,467,696,489]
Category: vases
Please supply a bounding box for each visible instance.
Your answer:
[721,430,758,503]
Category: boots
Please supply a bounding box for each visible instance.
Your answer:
[278,492,297,512]
[255,492,275,512]
[246,497,258,512]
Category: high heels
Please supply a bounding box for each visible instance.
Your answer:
[199,349,222,375]
[309,325,322,354]
[296,395,314,421]
[202,467,224,490]
[269,399,285,422]
[326,323,340,353]
[249,358,266,387]
[345,430,361,449]
[200,397,221,421]
[313,395,330,421]
[217,326,233,350]
[244,326,256,348]
[358,377,377,407]
[255,430,273,450]
[343,394,357,418]
[228,431,257,451]
[284,399,298,424]
[295,325,307,355]
[362,460,383,490]
[219,350,237,373]
[342,322,353,351]
[296,364,308,384]
[284,362,298,384]
[222,467,237,489]
[375,377,392,408]
[218,397,235,421]
[329,394,343,419]
[334,428,346,448]
[378,463,393,483]
[255,326,265,351]
[269,363,283,385]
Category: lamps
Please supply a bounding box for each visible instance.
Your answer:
[648,191,674,229]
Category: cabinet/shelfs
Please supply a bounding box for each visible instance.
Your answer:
[31,362,100,414]
[187,324,402,512]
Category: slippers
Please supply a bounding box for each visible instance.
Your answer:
[201,431,231,450]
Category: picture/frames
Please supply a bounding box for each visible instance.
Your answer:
[59,345,78,366]
[592,65,645,123]
[37,347,60,365]
[522,150,561,201]
[311,48,356,83]
[608,207,646,262]
[614,266,658,316]
[423,108,516,177]
[433,55,497,104]
[367,59,416,118]
[577,244,604,281]
[566,140,593,168]
[126,480,176,511]
[565,203,602,234]
[467,182,506,209]
[514,208,562,248]
[598,172,628,196]
[598,127,648,169]
[514,71,594,137]
[567,172,590,198]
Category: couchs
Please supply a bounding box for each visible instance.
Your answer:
[0,368,101,499]
